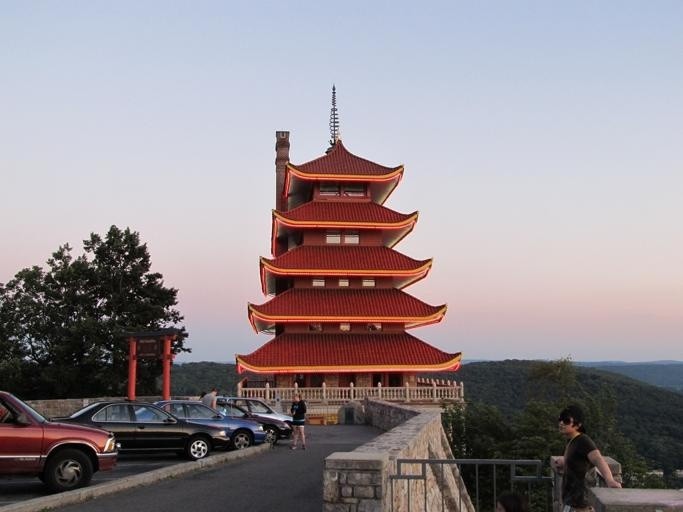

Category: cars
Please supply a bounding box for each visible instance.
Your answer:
[45,399,229,459]
[0,390,119,492]
[128,400,265,451]
[208,398,292,439]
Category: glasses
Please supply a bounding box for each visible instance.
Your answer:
[558,415,571,425]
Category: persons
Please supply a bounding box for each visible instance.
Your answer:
[198,392,207,400]
[289,393,308,450]
[557,405,624,511]
[201,388,219,410]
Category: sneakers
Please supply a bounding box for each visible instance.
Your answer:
[301,445,305,449]
[291,445,297,449]
